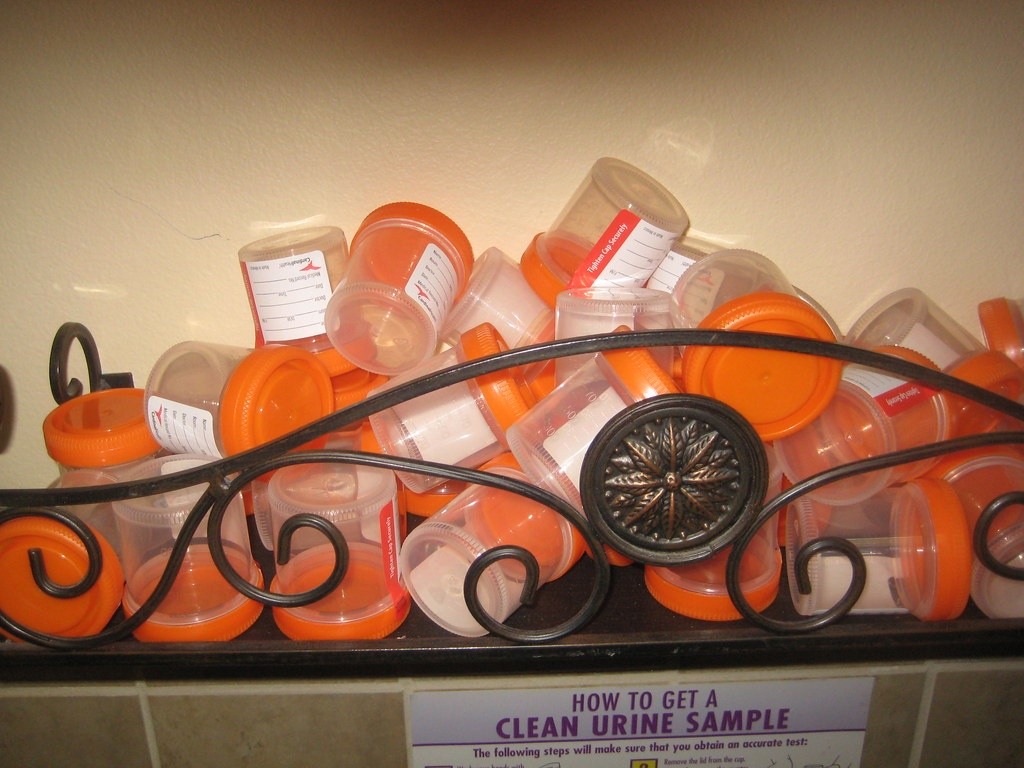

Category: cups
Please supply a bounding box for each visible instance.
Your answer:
[0,157,1024,642]
